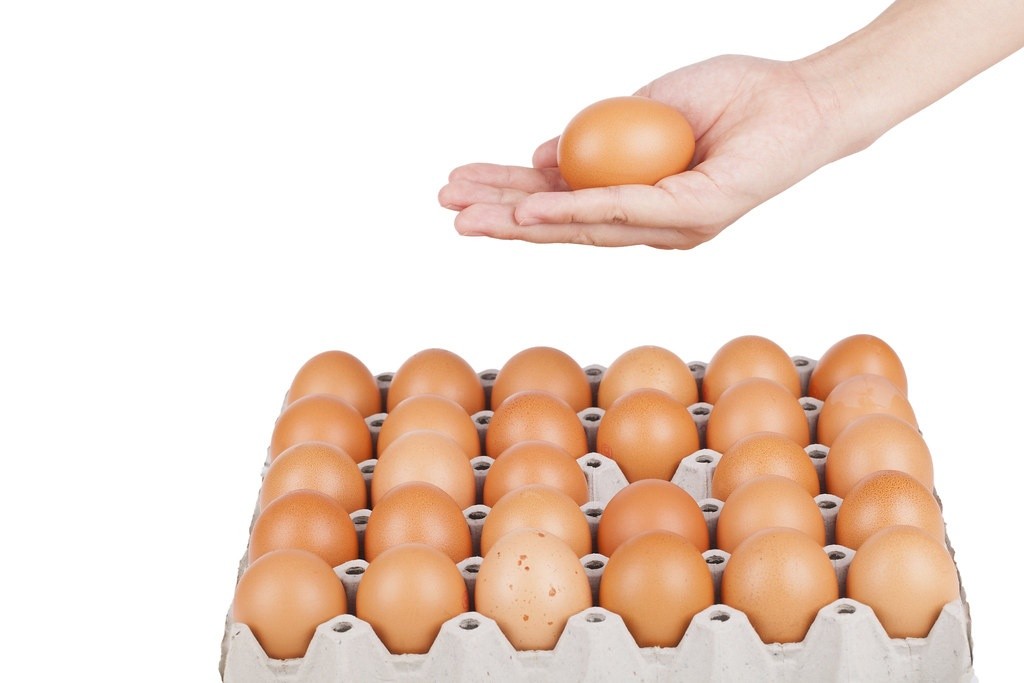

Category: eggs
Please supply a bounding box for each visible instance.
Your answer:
[556,96,694,189]
[232,334,960,659]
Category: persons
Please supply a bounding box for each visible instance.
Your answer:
[439,0,1024,250]
